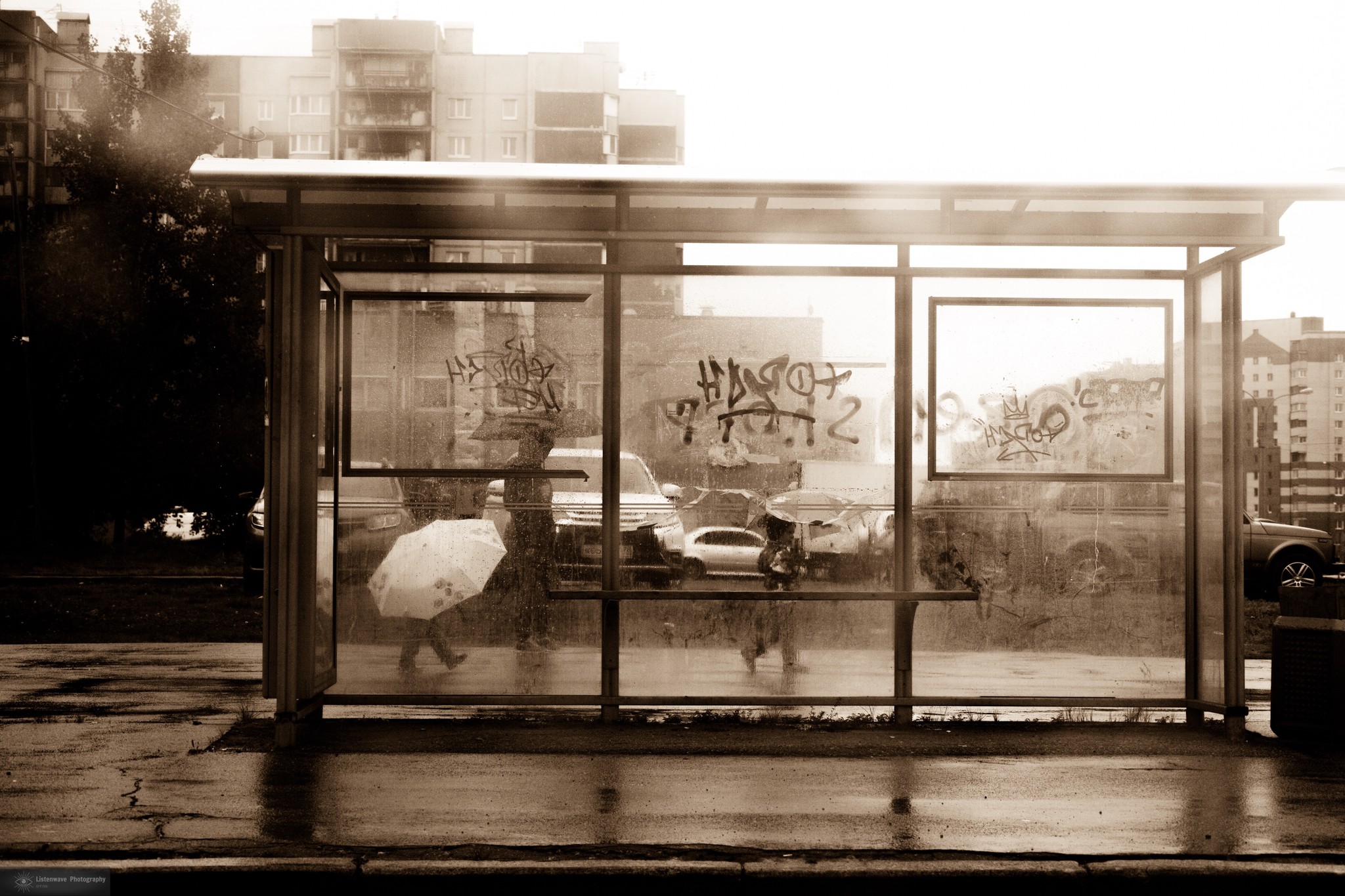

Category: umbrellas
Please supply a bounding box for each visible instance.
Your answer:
[743,483,872,556]
[368,518,508,622]
[467,403,604,443]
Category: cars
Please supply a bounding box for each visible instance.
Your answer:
[683,526,805,579]
[480,446,682,585]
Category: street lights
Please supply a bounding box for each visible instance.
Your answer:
[1243,387,1314,519]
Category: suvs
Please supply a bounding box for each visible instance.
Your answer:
[1011,475,1334,599]
[238,458,426,579]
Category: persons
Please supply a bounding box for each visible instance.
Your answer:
[396,615,467,682]
[740,512,812,675]
[503,432,565,658]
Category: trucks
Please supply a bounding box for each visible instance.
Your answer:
[795,457,940,580]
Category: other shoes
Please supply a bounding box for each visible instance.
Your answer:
[740,647,758,674]
[784,662,810,673]
[446,653,468,670]
